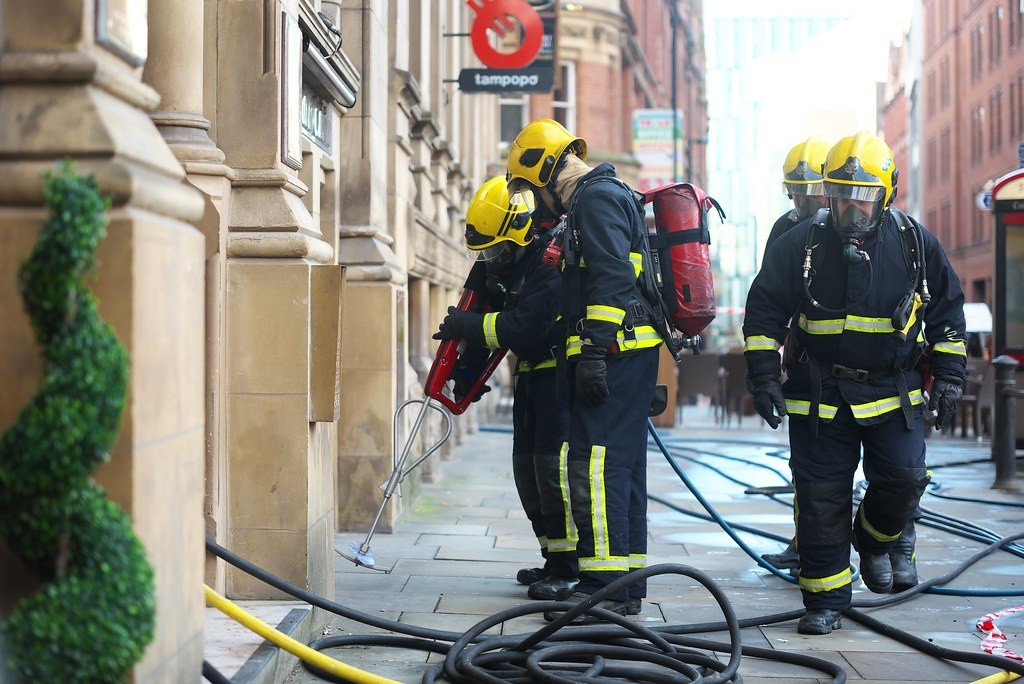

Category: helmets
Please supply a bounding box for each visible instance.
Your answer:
[781,138,830,199]
[820,133,898,208]
[466,175,535,251]
[505,120,587,186]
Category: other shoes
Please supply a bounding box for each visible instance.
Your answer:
[544,593,626,623]
[555,588,642,615]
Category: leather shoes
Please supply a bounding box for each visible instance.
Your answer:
[759,536,797,569]
[798,609,841,635]
[528,577,580,601]
[890,538,918,590]
[517,568,548,584]
[852,529,893,593]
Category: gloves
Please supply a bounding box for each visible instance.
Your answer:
[746,371,754,394]
[928,376,963,430]
[451,376,491,404]
[432,304,462,340]
[753,375,788,430]
[575,345,610,408]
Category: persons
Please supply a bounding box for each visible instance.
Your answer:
[431,175,580,599]
[742,132,969,635]
[506,119,667,625]
[757,137,932,594]
